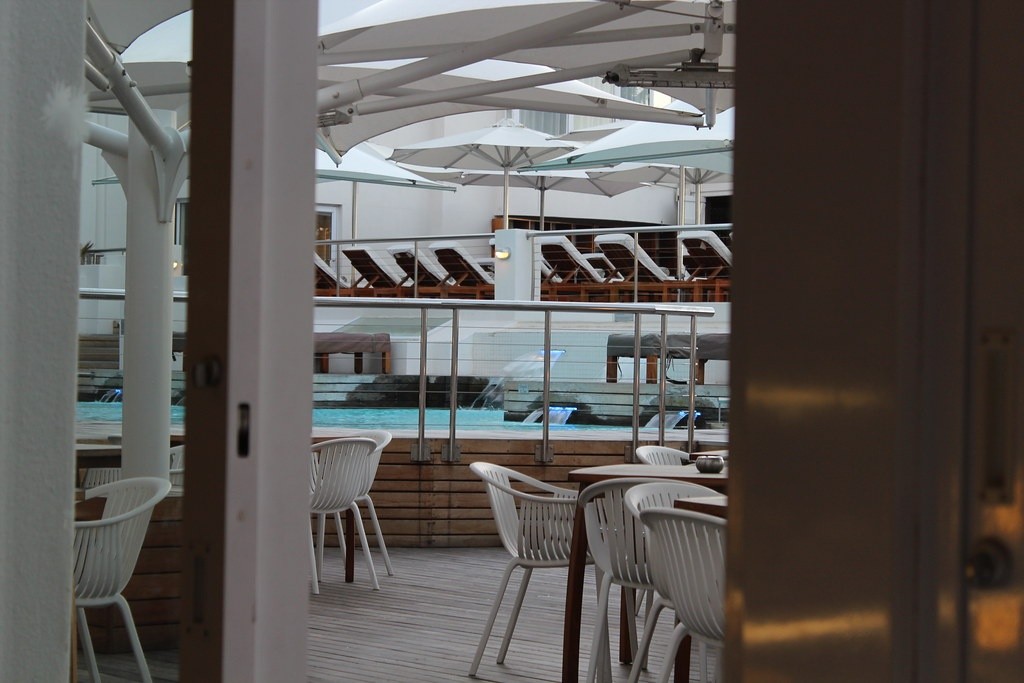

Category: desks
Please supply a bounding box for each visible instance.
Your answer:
[74,445,121,490]
[689,449,728,464]
[311,436,360,582]
[561,460,728,683]
[674,497,726,683]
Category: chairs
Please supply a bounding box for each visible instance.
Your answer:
[306,430,392,594]
[467,462,726,682]
[636,445,690,466]
[313,231,736,301]
[69,444,187,683]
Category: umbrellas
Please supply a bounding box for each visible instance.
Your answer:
[87,0,741,235]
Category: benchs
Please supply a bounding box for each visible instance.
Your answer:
[313,332,392,374]
[607,335,732,385]
